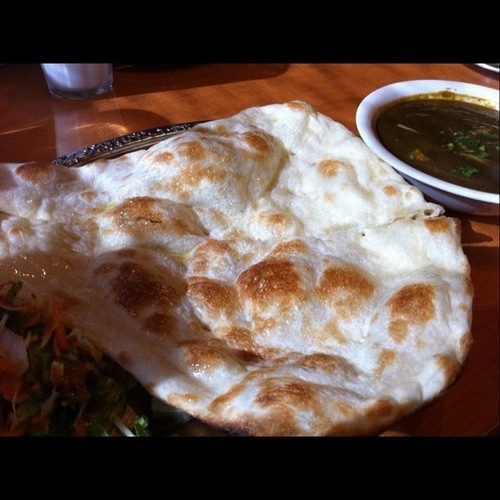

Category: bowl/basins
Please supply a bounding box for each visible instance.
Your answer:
[355,80,500,216]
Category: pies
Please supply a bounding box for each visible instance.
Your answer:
[0,98,474,437]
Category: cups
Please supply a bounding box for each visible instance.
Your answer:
[40,64,114,101]
